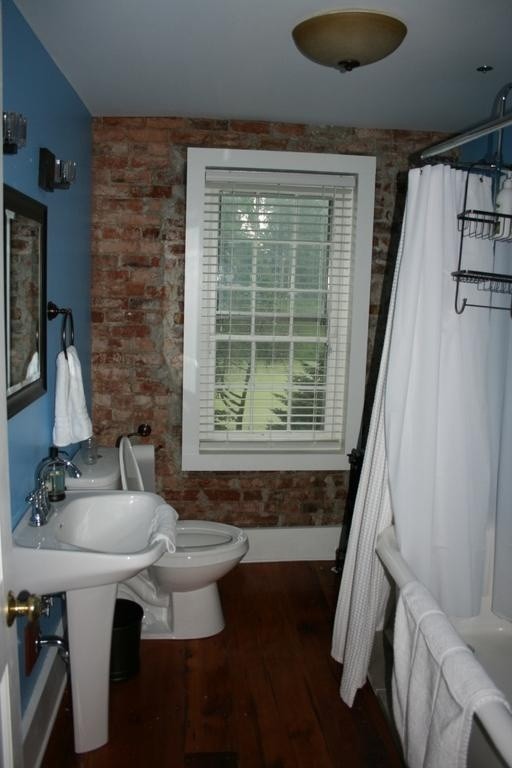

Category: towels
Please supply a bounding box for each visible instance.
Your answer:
[51,343,94,447]
[389,580,510,768]
[25,352,40,380]
[147,503,181,555]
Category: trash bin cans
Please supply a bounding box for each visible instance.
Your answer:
[109,598,144,682]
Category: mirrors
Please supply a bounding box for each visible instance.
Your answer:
[2,182,47,421]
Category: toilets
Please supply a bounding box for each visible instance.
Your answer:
[64,435,250,641]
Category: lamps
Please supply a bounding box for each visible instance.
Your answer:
[289,6,410,73]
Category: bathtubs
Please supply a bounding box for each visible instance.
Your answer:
[374,524,512,768]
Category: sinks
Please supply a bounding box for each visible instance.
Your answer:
[12,489,176,593]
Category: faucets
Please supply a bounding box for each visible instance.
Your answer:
[22,455,82,526]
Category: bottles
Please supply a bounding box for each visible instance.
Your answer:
[79,437,98,465]
